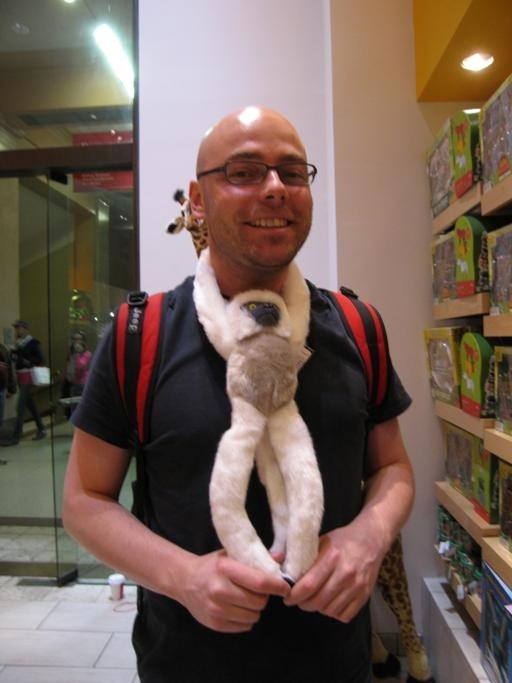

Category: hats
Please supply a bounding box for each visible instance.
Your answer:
[13,320,30,328]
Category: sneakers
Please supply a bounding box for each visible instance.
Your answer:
[32,429,47,440]
[1,436,19,445]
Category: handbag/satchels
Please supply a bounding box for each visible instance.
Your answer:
[18,366,50,387]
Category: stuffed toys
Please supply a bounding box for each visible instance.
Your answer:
[193,245,326,597]
[166,187,432,683]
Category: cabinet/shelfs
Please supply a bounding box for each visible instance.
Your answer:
[409,0,512,683]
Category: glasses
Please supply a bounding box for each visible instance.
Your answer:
[197,158,316,186]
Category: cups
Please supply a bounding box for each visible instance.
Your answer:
[109,574,126,601]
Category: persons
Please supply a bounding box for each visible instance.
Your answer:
[0,343,17,464]
[9,318,55,440]
[61,104,416,682]
[61,330,93,420]
